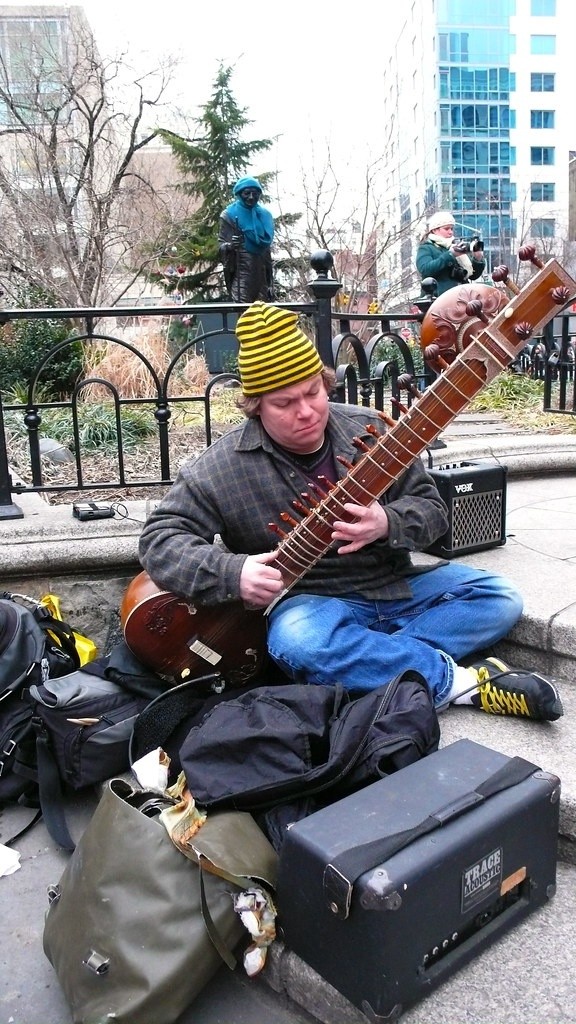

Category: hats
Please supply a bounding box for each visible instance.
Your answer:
[428,212,455,231]
[235,301,323,397]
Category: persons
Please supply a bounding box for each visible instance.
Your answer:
[416,212,486,299]
[517,335,576,383]
[218,176,274,302]
[138,298,564,724]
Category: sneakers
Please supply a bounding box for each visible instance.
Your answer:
[469,656,563,721]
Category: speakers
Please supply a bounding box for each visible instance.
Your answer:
[422,461,509,559]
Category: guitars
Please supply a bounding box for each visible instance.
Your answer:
[126,242,576,677]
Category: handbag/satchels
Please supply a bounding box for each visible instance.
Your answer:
[42,778,281,1024]
[179,670,441,856]
[0,590,190,853]
